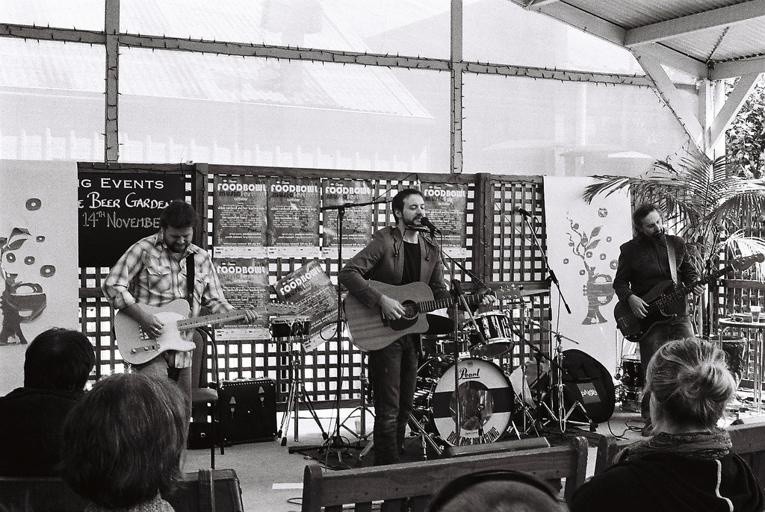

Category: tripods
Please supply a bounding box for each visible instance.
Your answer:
[275,351,332,444]
[536,321,597,436]
[288,217,366,467]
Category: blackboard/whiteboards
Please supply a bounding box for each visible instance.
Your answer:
[78,169,185,268]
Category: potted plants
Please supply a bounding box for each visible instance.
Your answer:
[582,147,765,397]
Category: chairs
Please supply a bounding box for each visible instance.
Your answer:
[595,425,765,496]
[192,327,224,470]
[0,469,245,511]
[301,436,588,512]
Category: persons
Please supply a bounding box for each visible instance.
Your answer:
[569,338,765,512]
[613,205,706,436]
[338,189,496,466]
[0,327,95,478]
[102,203,258,472]
[61,373,191,512]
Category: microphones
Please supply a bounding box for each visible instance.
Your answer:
[512,204,532,220]
[420,216,441,236]
[416,174,420,191]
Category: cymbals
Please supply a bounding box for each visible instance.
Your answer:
[424,312,457,338]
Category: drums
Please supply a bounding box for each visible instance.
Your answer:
[408,350,517,447]
[270,314,312,344]
[461,309,515,358]
[433,329,474,358]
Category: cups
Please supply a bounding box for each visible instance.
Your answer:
[750,305,762,325]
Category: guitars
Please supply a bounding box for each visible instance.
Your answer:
[110,294,301,365]
[613,252,765,343]
[341,277,524,352]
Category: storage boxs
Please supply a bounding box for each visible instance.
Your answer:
[209,377,279,445]
